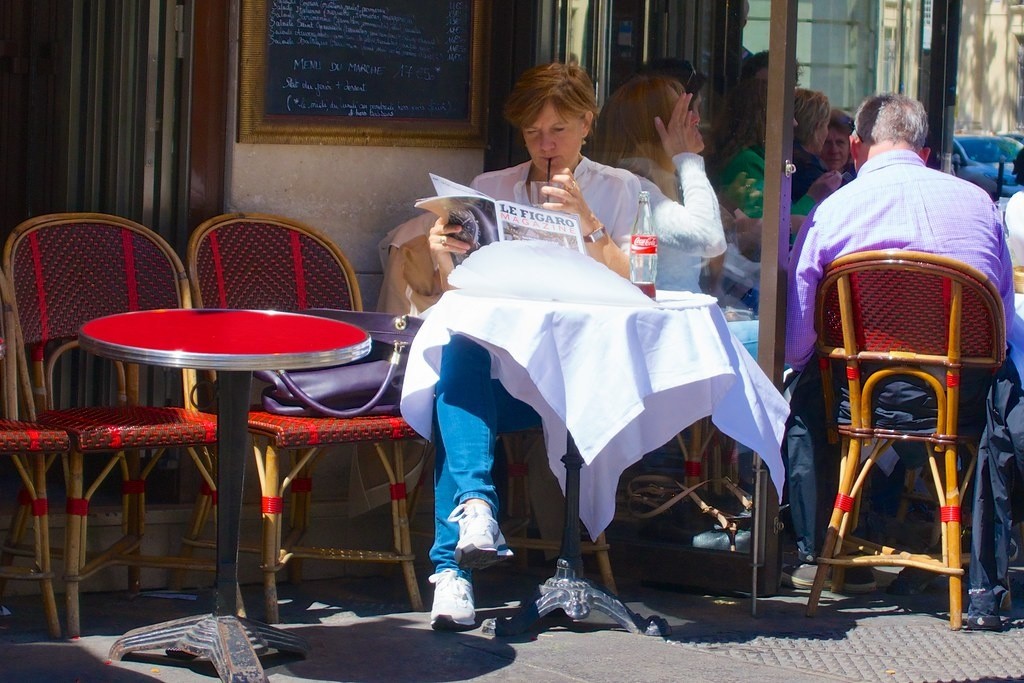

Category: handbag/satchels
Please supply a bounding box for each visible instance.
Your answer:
[628,472,757,594]
[253,307,425,419]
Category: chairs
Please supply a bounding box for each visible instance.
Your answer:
[168,212,759,625]
[803,250,1008,632]
[1,213,246,639]
[0,269,70,639]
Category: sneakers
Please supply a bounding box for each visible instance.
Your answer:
[447,503,515,566]
[428,569,476,629]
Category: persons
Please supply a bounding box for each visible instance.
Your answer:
[428,63,643,631]
[600,53,1024,595]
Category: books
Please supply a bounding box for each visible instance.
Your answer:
[413,173,585,255]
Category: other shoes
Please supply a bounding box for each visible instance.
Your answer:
[779,558,880,593]
[889,565,944,596]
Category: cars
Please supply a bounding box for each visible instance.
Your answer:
[953,132,1024,201]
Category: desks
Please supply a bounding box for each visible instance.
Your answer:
[399,289,791,639]
[80,308,373,683]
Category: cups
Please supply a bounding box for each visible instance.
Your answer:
[1013,266,1024,293]
[530,181,566,212]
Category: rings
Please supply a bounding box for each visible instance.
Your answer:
[441,237,447,244]
[568,181,576,191]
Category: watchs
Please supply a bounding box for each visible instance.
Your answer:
[583,225,607,244]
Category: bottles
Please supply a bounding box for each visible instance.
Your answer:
[631,192,658,301]
[698,254,725,296]
